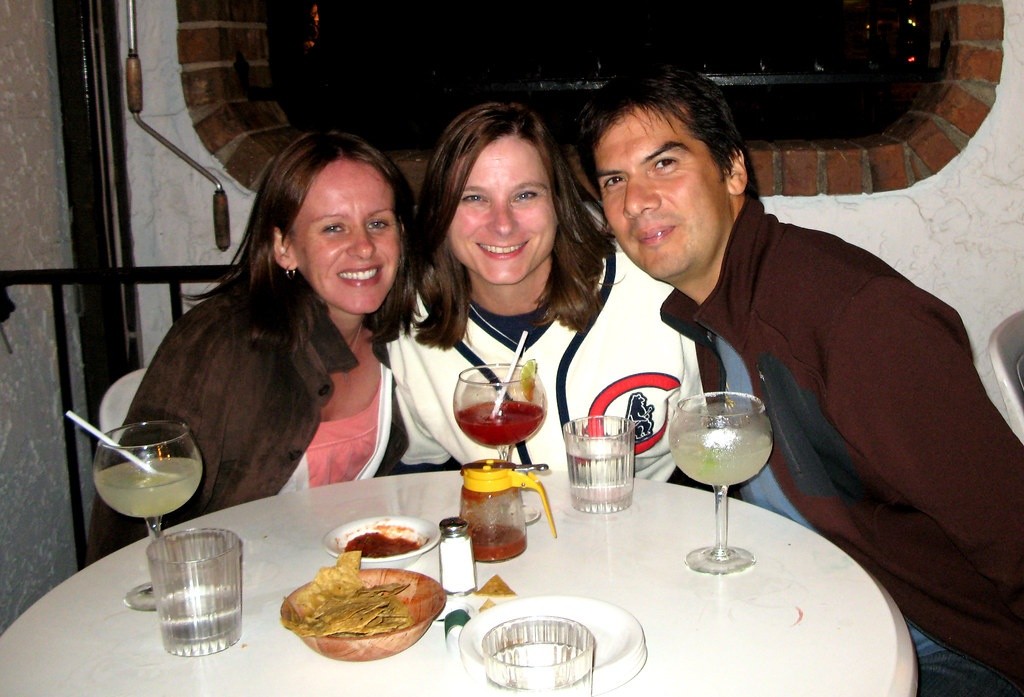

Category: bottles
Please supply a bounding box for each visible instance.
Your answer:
[439,517,478,596]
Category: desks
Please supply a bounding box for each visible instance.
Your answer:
[0,470,921,697]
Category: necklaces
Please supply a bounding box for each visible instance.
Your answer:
[469,303,552,355]
[349,321,365,354]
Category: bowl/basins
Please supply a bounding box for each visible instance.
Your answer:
[323,515,441,570]
[281,568,447,662]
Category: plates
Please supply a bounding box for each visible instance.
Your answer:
[458,595,647,697]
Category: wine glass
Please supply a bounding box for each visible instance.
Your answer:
[93,419,203,612]
[453,363,548,526]
[669,392,773,574]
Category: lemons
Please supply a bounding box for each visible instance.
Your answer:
[520,360,537,402]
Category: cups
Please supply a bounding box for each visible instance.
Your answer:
[458,459,557,564]
[146,528,243,656]
[483,615,597,697]
[563,416,636,514]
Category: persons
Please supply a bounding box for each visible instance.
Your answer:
[382,100,699,477]
[576,65,1024,696]
[86,128,420,570]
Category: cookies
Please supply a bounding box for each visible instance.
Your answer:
[281,551,413,639]
[478,599,495,612]
[475,574,515,596]
